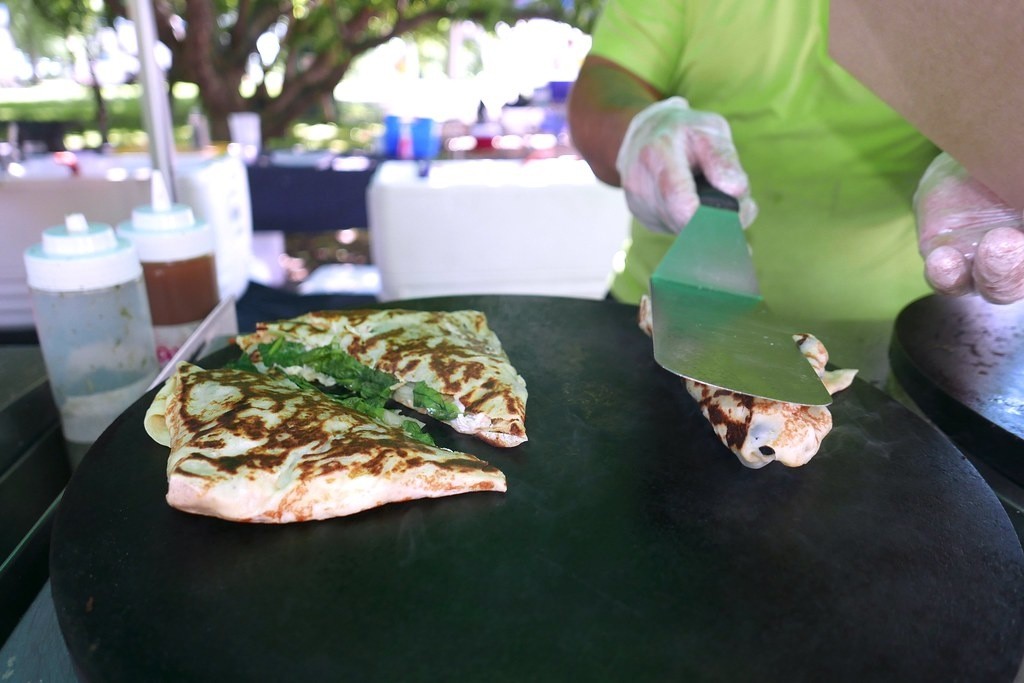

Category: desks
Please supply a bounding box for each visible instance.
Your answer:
[368,159,631,299]
[242,150,382,231]
[0,150,250,329]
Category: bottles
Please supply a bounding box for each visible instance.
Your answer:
[23,212,162,443]
[116,170,221,365]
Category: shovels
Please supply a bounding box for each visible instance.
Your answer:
[647,172,837,407]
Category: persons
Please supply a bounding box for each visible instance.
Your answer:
[568,0,1024,419]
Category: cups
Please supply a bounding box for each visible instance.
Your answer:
[226,111,469,161]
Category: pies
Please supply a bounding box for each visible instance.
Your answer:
[637,295,859,468]
[144,359,507,523]
[235,310,531,448]
